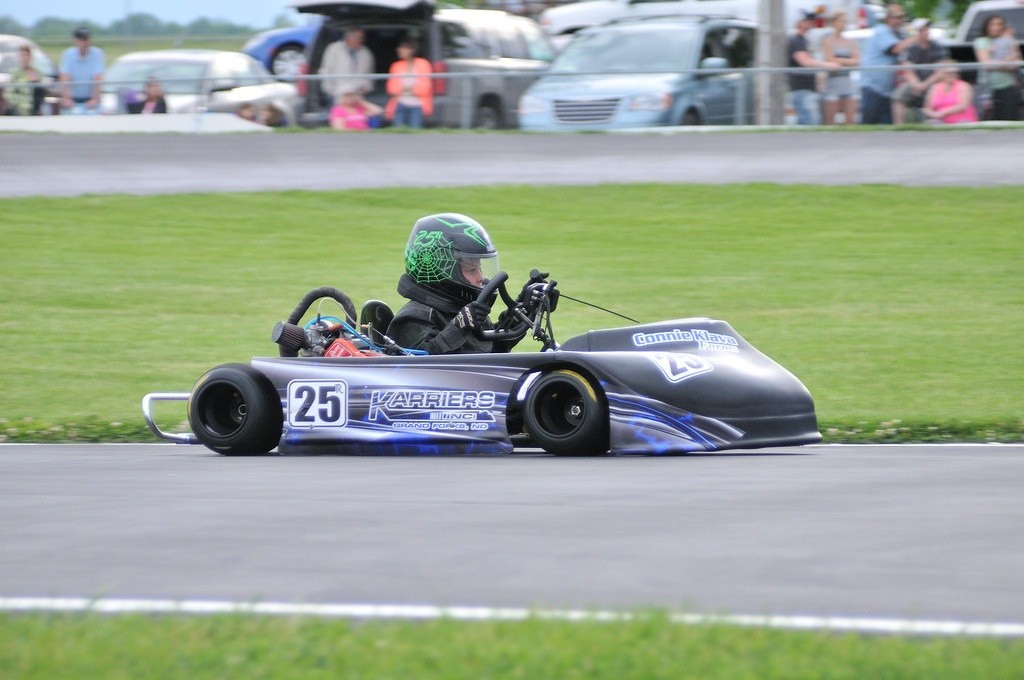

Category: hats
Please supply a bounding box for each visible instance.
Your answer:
[911,17,931,30]
[795,9,817,21]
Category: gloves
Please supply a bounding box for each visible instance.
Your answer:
[450,301,491,330]
[530,284,548,302]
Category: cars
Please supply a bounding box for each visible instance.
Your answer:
[101,48,306,132]
[242,18,324,84]
[0,33,60,88]
[958,0,1024,45]
[519,18,785,128]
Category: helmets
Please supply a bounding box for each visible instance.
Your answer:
[405,213,500,308]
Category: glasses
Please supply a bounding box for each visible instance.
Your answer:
[890,13,908,20]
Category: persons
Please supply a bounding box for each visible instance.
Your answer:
[124,74,169,117]
[382,210,558,369]
[1,45,49,119]
[786,1,1024,125]
[56,26,107,119]
[230,96,298,127]
[316,21,437,131]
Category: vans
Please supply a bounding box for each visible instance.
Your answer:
[284,2,556,130]
